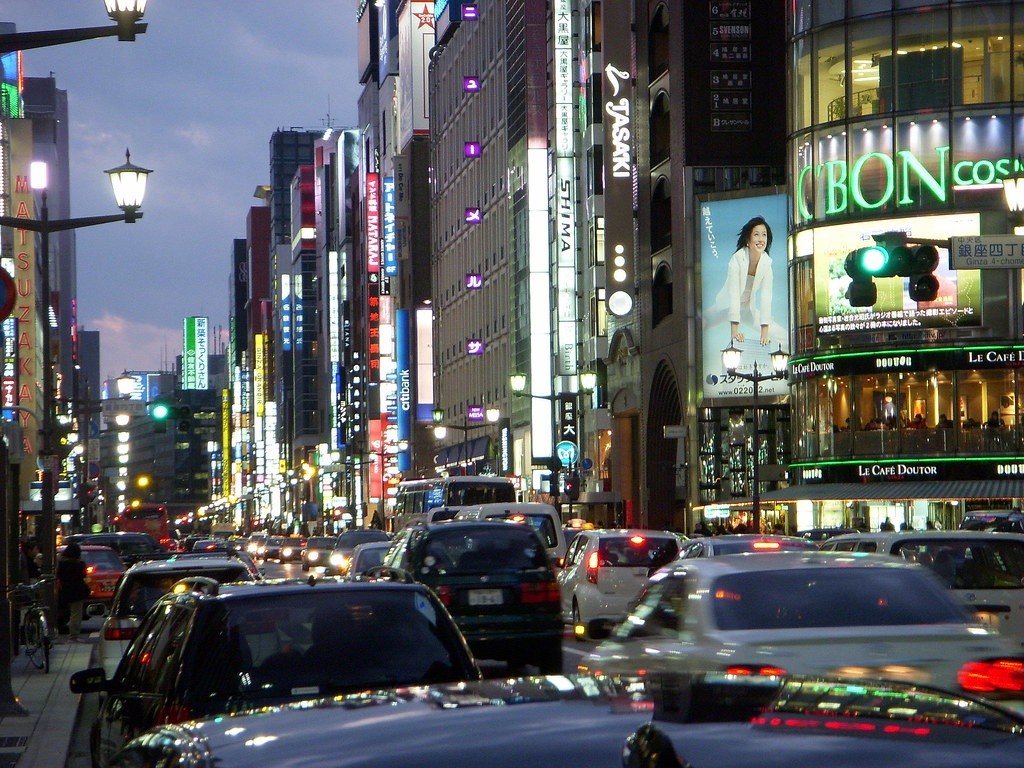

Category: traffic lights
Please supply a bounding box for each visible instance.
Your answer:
[565,479,579,500]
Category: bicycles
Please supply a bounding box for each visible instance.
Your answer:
[7,574,57,673]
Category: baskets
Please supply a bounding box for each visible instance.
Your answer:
[11,591,33,609]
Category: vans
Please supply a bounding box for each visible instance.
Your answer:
[456,502,568,571]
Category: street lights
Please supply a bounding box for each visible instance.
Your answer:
[432,402,501,477]
[1,147,155,639]
[86,402,173,533]
[508,369,598,507]
[723,339,794,536]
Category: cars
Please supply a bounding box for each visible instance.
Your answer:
[112,668,1024,768]
[574,552,1024,698]
[55,532,265,708]
[561,508,1024,651]
[268,528,395,575]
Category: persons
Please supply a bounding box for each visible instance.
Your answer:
[900,522,920,556]
[985,410,1006,453]
[839,414,954,455]
[880,517,894,532]
[694,519,796,537]
[702,215,789,351]
[20,536,89,645]
[926,521,936,531]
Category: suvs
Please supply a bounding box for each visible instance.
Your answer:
[385,518,560,670]
[70,573,484,768]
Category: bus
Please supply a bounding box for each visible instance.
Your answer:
[120,502,170,532]
[391,477,518,518]
